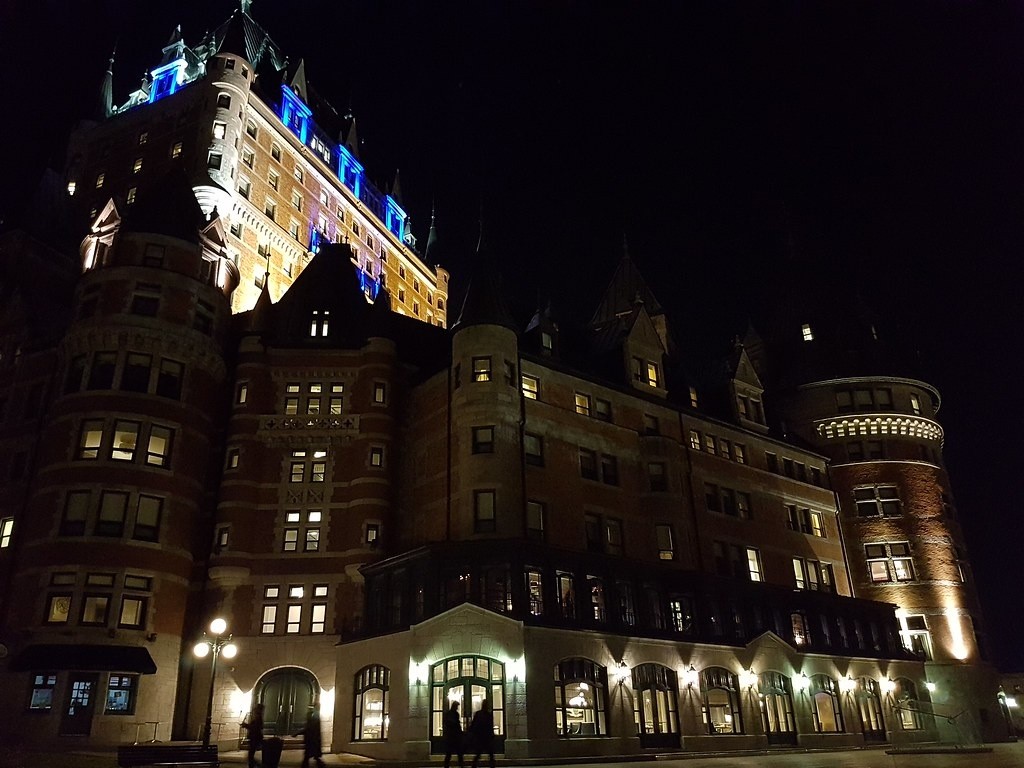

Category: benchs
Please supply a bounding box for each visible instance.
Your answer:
[567,721,595,734]
[116,744,220,768]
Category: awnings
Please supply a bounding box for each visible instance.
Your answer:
[31,643,157,675]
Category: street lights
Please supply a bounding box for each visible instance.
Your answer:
[193,618,237,763]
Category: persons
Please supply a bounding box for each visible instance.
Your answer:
[470,700,496,768]
[292,710,326,768]
[241,704,264,768]
[443,701,465,768]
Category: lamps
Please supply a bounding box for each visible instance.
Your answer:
[887,676,894,695]
[150,633,158,641]
[748,668,756,690]
[800,671,811,692]
[845,674,856,695]
[108,629,117,637]
[618,662,628,685]
[687,663,697,688]
